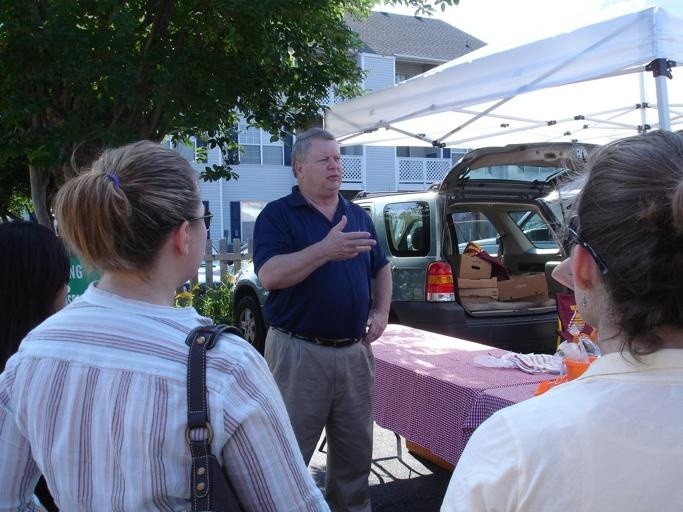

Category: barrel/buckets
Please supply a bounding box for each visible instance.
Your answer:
[562,355,597,382]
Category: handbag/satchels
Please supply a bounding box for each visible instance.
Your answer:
[186,322,245,512]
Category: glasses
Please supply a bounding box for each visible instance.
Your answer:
[568,214,608,275]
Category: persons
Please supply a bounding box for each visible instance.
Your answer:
[397,217,428,253]
[439,130,683,512]
[0,139,333,511]
[252,128,394,511]
[0,219,69,510]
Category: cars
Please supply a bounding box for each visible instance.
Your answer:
[229,141,603,357]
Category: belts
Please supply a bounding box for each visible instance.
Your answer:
[277,328,362,348]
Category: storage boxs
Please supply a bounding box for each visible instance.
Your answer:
[448,252,549,310]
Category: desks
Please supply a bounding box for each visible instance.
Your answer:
[317,322,564,466]
